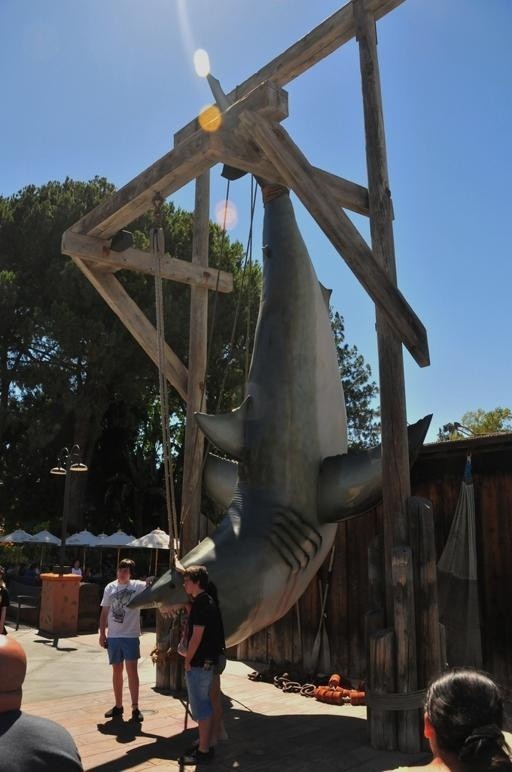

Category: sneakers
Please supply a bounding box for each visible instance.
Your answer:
[103,704,125,718]
[176,747,211,766]
[184,744,215,759]
[131,708,145,722]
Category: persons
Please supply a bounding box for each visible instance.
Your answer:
[184,581,229,747]
[66,559,83,581]
[98,558,159,722]
[180,564,223,764]
[384,669,512,772]
[0,564,83,772]
[0,566,11,635]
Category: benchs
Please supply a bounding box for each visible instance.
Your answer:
[6,579,41,630]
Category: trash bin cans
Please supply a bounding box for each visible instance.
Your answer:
[38,573,82,639]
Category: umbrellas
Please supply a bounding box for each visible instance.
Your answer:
[126,526,180,577]
[23,528,61,562]
[56,528,97,573]
[89,528,135,579]
[0,526,32,543]
